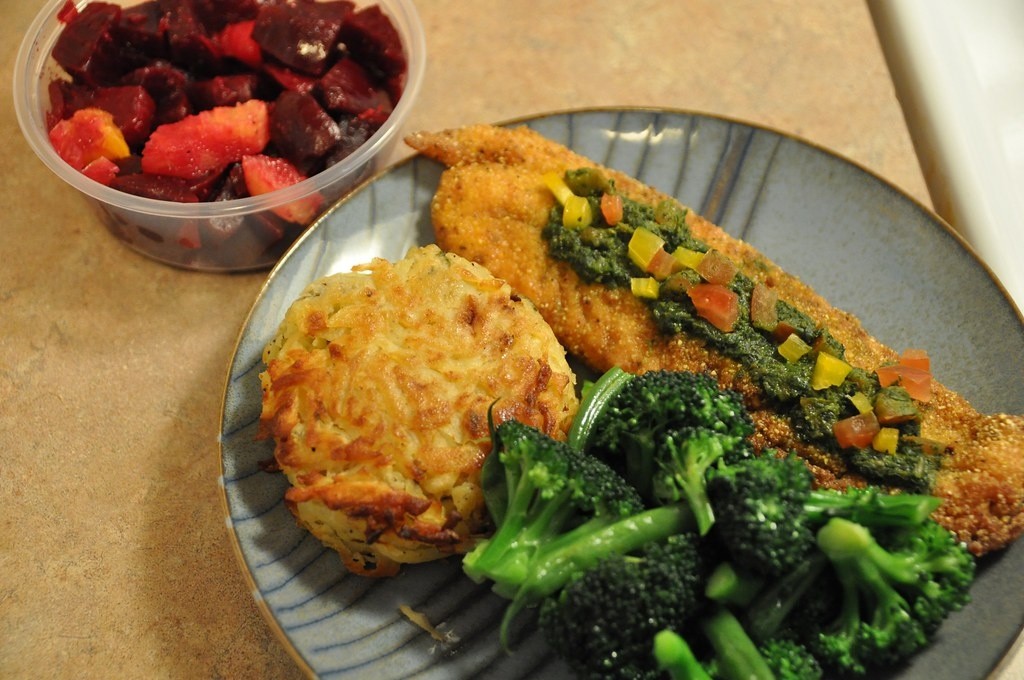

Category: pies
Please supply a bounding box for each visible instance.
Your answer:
[256,244,577,581]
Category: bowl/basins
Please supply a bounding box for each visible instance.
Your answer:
[13,0,428,273]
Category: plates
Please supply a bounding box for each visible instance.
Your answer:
[217,104,1024,680]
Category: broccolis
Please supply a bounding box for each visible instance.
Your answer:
[459,366,978,680]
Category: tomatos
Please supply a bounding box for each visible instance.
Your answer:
[44,99,327,225]
